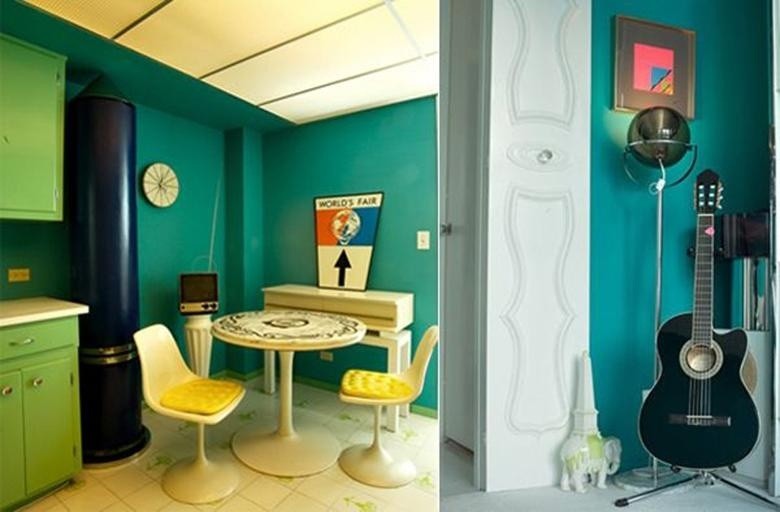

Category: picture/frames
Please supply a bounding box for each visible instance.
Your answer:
[612,14,696,120]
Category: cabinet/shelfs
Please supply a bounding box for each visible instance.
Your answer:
[0,31,68,222]
[1,295,90,512]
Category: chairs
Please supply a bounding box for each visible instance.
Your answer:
[340,326,438,489]
[134,324,246,505]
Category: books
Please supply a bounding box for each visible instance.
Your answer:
[730,257,771,333]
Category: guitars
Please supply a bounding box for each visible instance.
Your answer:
[637,169,760,470]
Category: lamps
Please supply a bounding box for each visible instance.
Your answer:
[621,106,698,191]
